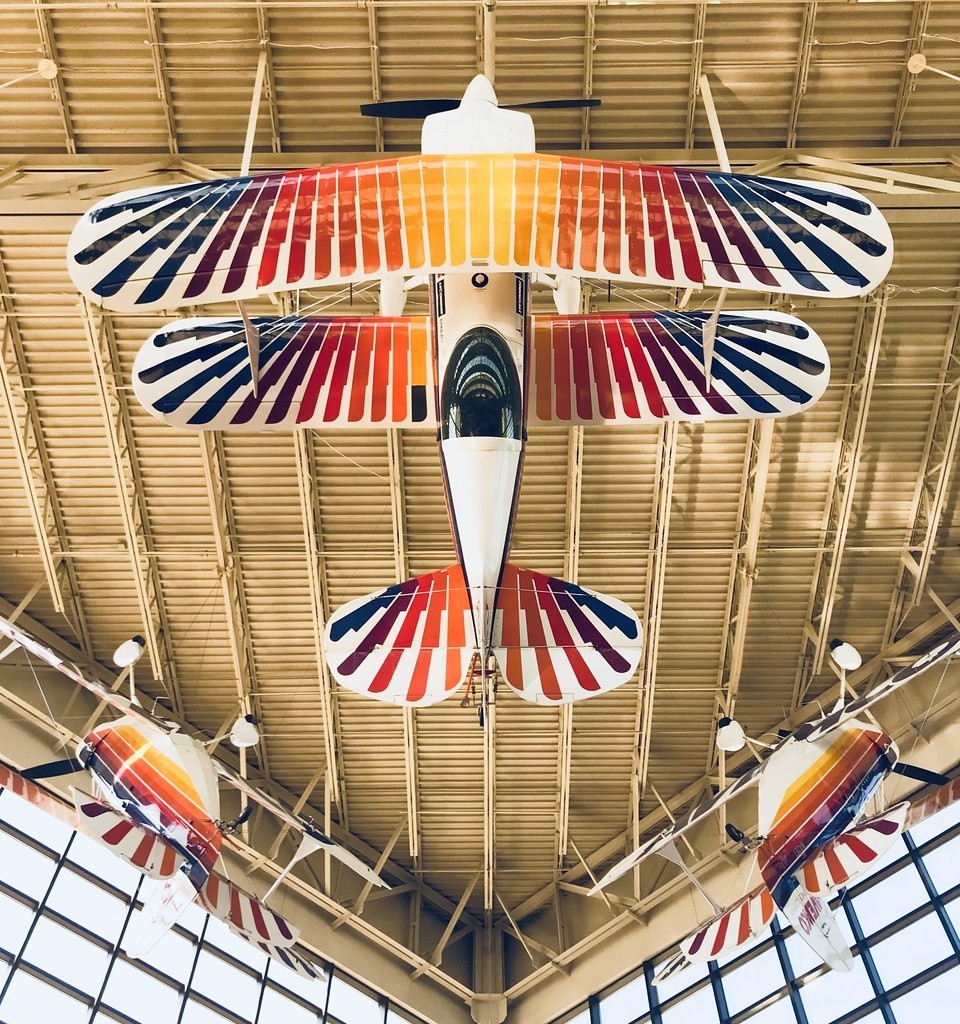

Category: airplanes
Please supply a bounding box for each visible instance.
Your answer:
[588,635,960,987]
[65,73,895,707]
[0,614,393,983]
